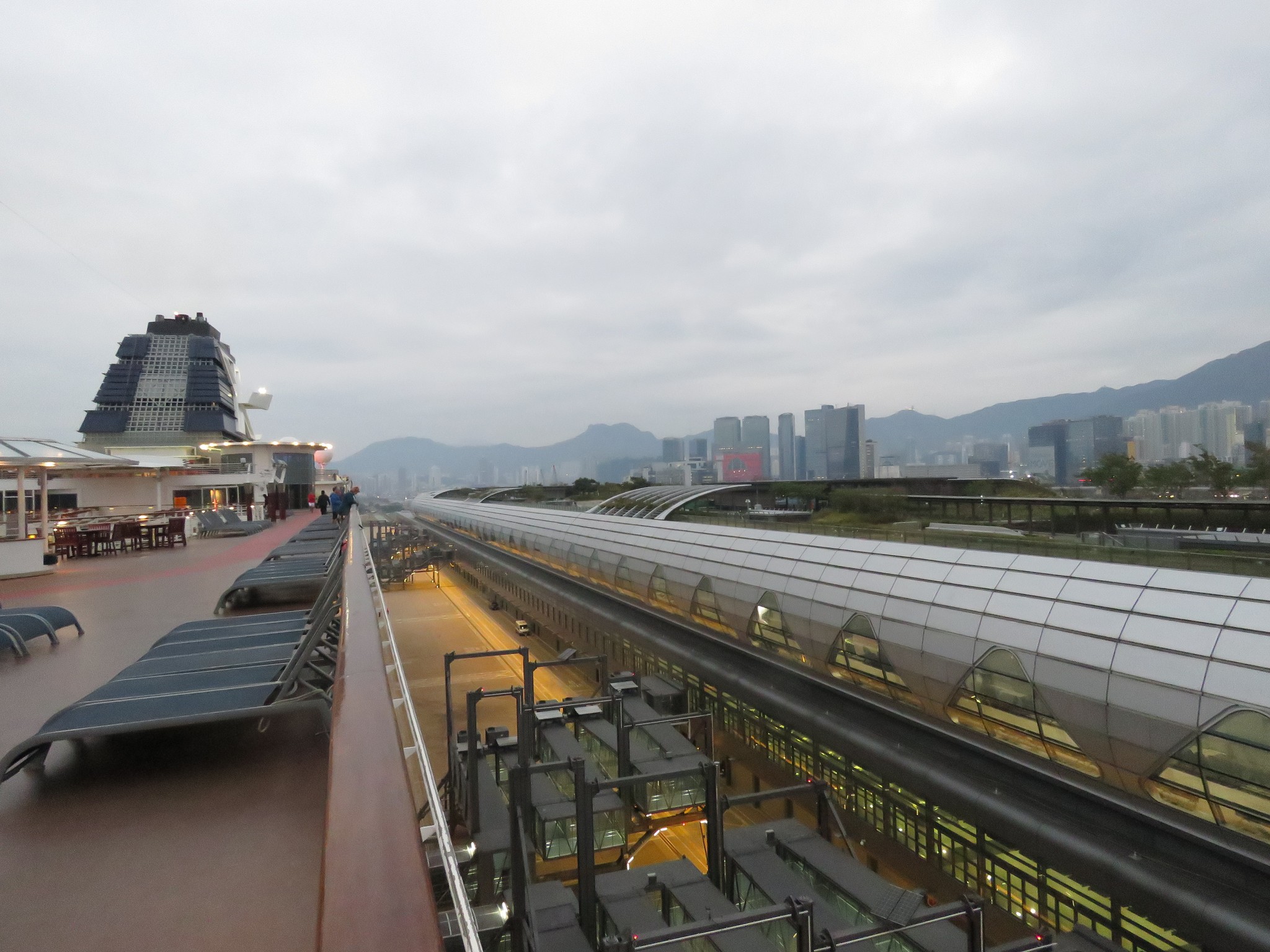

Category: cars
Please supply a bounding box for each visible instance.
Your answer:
[488,602,499,610]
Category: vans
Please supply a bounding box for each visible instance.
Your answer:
[515,620,530,636]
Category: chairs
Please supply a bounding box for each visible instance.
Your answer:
[49,526,78,560]
[117,521,144,551]
[154,517,188,548]
[94,522,128,558]
[194,504,350,615]
[79,522,112,556]
[0,547,348,785]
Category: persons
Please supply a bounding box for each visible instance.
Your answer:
[329,486,343,524]
[307,491,318,513]
[339,486,360,520]
[316,490,331,515]
[262,494,269,515]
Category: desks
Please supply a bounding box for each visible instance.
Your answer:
[62,528,110,560]
[137,522,175,549]
